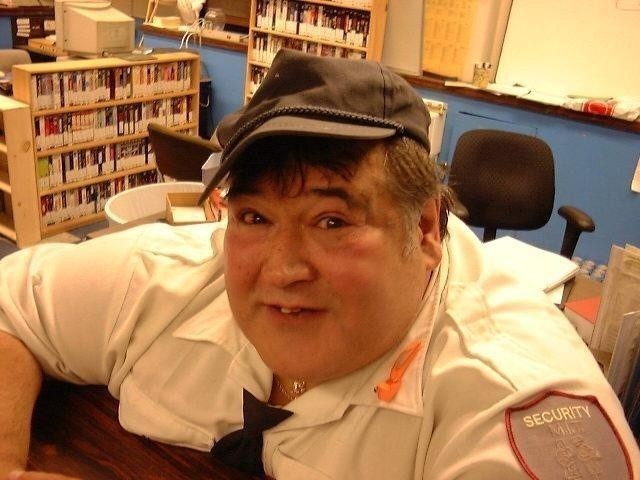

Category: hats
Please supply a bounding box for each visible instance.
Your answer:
[197,49,430,203]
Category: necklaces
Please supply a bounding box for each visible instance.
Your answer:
[271,376,305,403]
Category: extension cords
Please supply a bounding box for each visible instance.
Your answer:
[198,28,243,43]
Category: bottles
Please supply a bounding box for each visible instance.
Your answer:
[472,62,492,91]
[203,7,227,33]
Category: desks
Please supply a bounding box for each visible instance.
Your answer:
[26,376,268,480]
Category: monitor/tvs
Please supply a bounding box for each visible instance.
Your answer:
[54,0,136,58]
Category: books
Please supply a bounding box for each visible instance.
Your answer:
[16,18,194,226]
[247,0,371,103]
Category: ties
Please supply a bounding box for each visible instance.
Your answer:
[209,387,293,478]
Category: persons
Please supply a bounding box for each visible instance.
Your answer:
[0,49,639,480]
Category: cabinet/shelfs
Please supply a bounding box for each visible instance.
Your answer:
[0,49,202,251]
[244,0,388,104]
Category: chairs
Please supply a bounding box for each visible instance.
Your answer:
[445,129,597,262]
